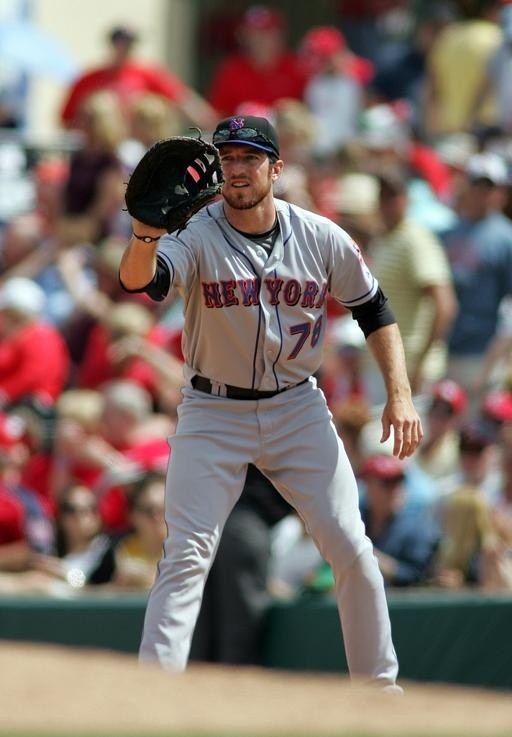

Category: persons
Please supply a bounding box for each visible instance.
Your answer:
[117,115,424,696]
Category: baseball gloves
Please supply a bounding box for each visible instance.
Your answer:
[124,138,226,236]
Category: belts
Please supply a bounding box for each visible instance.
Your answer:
[190,374,309,401]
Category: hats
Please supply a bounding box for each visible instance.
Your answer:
[212,113,281,159]
